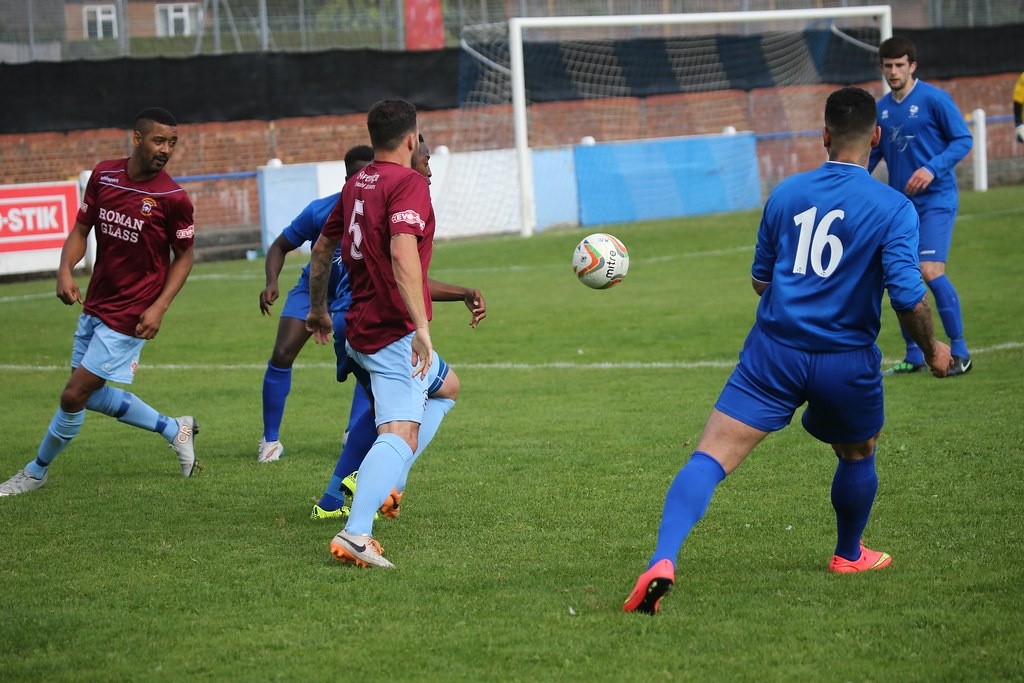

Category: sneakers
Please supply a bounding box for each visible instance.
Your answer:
[342,431,350,448]
[310,504,378,521]
[883,358,928,376]
[257,436,284,463]
[623,558,674,615]
[339,471,357,501]
[829,542,892,574]
[168,416,199,478]
[0,469,48,496]
[931,355,972,378]
[329,530,395,569]
[379,487,403,521]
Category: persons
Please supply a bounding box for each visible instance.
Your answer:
[258,142,373,463]
[868,36,975,377]
[625,86,954,616]
[305,97,487,566]
[1012,72,1024,142]
[0,106,199,496]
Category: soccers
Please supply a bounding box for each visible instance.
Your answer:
[571,232,630,290]
